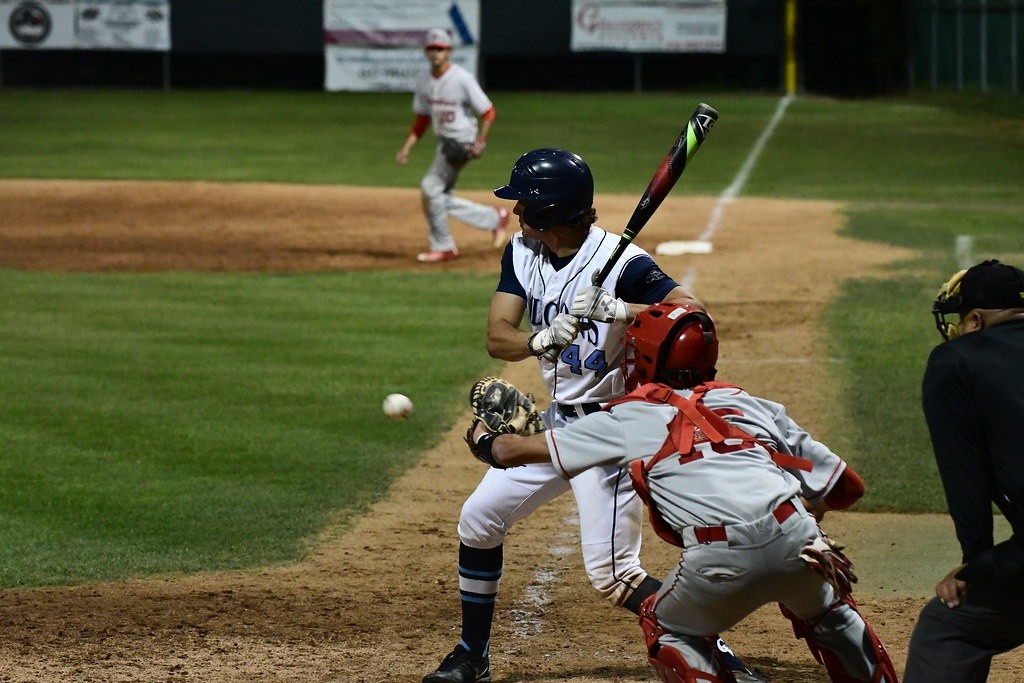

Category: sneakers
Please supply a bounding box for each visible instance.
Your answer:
[720,654,767,683]
[422,644,491,683]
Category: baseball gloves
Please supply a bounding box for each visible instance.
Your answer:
[467,377,547,436]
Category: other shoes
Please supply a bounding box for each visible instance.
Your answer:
[417,248,460,262]
[492,207,512,250]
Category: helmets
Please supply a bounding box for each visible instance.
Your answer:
[933,258,1024,313]
[494,148,594,231]
[620,302,719,389]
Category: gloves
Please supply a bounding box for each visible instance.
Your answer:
[800,536,858,594]
[572,286,628,323]
[532,313,582,350]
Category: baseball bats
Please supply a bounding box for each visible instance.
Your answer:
[542,102,719,364]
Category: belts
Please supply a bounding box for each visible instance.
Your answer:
[694,501,798,544]
[557,402,602,418]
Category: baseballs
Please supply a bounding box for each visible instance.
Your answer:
[382,393,413,423]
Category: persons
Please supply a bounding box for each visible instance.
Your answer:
[423,147,766,683]
[396,30,512,262]
[899,258,1024,683]
[464,302,900,683]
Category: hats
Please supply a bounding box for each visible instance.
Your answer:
[424,28,453,49]
